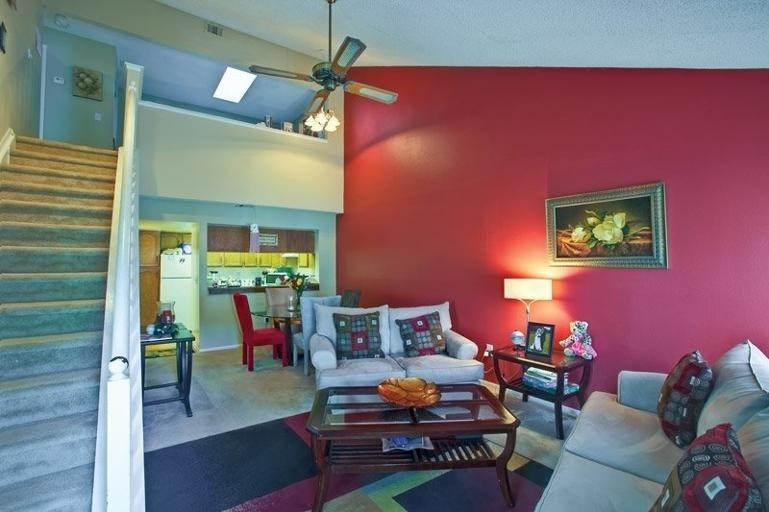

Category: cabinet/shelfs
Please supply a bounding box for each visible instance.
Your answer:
[136,266,161,333]
[138,230,162,268]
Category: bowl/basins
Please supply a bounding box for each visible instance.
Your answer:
[375,378,442,411]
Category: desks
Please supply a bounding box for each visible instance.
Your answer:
[487,343,595,442]
[139,321,196,427]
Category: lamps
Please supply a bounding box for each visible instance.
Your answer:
[300,99,341,132]
[501,276,554,339]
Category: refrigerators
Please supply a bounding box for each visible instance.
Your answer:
[159,253,191,328]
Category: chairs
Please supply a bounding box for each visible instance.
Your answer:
[230,286,366,371]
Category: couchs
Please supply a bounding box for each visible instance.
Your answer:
[527,337,768,510]
[307,299,490,428]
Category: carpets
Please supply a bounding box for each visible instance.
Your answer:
[139,404,559,512]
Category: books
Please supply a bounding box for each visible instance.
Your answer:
[140,333,172,342]
[521,366,569,392]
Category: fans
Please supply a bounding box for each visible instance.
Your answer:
[249,0,405,132]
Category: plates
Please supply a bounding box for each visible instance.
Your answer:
[381,436,434,453]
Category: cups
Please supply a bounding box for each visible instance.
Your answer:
[264,115,272,129]
[255,277,261,285]
[146,326,154,335]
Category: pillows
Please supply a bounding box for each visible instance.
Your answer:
[655,349,716,452]
[642,422,767,512]
[331,310,388,360]
[393,310,450,362]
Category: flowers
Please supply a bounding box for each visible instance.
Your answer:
[568,206,632,252]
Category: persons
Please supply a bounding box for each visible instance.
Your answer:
[529,327,547,351]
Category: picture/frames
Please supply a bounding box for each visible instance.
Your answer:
[544,180,669,272]
[520,320,555,359]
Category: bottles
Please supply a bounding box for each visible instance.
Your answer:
[160,302,173,328]
